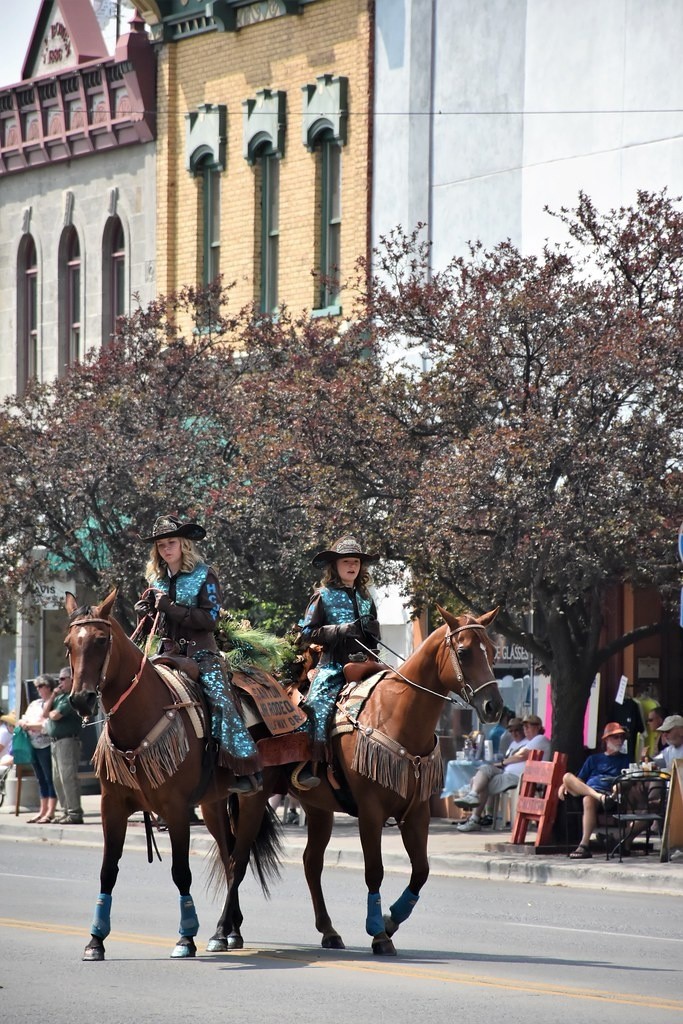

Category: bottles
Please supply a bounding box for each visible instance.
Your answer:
[475,743,482,759]
[485,740,494,762]
[464,739,472,761]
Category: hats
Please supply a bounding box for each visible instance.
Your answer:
[312,536,380,569]
[520,716,542,725]
[655,715,683,731]
[136,515,206,544]
[601,722,625,739]
[506,718,523,728]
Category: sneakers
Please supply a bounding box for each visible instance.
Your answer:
[453,793,480,808]
[457,820,481,831]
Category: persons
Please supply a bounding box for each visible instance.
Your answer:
[558,722,630,858]
[604,707,683,855]
[15,674,58,824]
[42,667,84,824]
[134,515,263,794]
[454,707,551,831]
[0,708,15,776]
[295,535,379,789]
[269,794,299,824]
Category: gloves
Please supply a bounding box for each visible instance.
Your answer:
[364,620,382,640]
[154,593,188,624]
[323,623,361,648]
[134,600,152,636]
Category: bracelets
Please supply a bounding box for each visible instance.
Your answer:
[501,761,505,766]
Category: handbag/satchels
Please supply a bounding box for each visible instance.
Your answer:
[12,726,36,764]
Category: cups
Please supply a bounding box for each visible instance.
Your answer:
[456,752,464,761]
[629,763,638,773]
[475,734,483,744]
[494,753,503,762]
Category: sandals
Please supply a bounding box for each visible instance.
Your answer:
[570,844,592,858]
[602,797,614,811]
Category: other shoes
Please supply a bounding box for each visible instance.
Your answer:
[286,812,299,824]
[227,772,264,792]
[479,815,493,825]
[27,816,42,823]
[37,815,53,823]
[50,814,67,824]
[59,816,83,824]
[290,761,321,791]
[595,833,630,856]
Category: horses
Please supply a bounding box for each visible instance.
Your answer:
[62,588,281,961]
[206,602,503,955]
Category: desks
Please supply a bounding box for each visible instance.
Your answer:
[441,758,492,818]
[601,776,671,855]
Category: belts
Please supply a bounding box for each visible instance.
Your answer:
[50,733,77,743]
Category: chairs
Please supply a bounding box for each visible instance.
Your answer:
[562,770,670,863]
[491,783,543,832]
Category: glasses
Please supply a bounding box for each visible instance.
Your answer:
[646,718,653,723]
[59,677,70,682]
[35,684,46,689]
